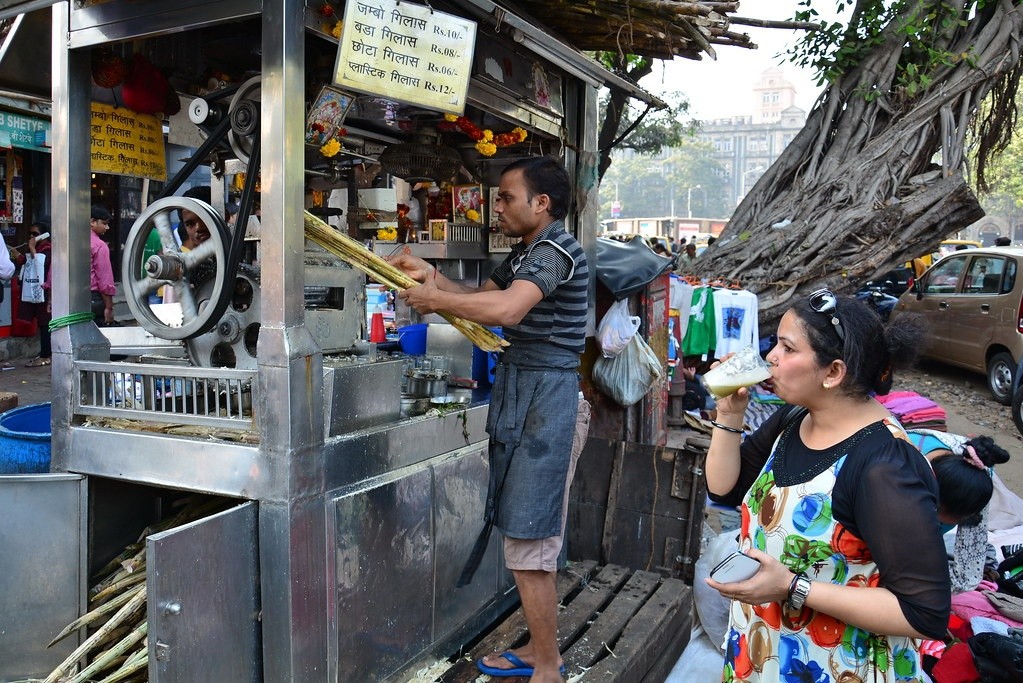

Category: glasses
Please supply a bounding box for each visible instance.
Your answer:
[29,230,40,237]
[809,286,845,341]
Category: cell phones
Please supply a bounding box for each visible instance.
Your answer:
[710,551,762,585]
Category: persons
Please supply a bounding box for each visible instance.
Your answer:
[704,287,1011,683]
[366,156,590,683]
[0,228,16,302]
[41,206,116,362]
[23,223,52,367]
[608,234,718,261]
[162,183,262,303]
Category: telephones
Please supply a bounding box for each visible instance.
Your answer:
[34,232,50,241]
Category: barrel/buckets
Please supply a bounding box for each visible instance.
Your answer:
[397,324,428,355]
[0,399,53,475]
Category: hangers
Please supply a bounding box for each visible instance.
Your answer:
[681,274,743,290]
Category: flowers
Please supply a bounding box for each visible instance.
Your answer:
[310,123,346,157]
[443,112,529,156]
[467,209,480,223]
[377,227,397,240]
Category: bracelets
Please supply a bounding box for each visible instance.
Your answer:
[710,419,745,434]
[787,576,811,610]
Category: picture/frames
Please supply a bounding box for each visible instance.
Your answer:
[305,86,357,147]
[489,186,523,253]
[448,181,484,225]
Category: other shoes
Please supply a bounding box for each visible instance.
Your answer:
[26,357,51,366]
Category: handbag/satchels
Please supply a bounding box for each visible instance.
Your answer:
[596,296,642,359]
[21,252,46,304]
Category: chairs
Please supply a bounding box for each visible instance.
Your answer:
[977,274,1000,293]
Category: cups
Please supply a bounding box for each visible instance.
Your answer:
[702,344,773,400]
[320,351,447,392]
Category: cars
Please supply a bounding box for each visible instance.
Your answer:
[888,246,1023,406]
[852,239,982,326]
[605,231,720,258]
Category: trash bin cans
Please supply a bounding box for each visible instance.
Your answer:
[0,402,51,474]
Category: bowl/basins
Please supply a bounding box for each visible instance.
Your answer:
[399,393,430,417]
[405,375,447,398]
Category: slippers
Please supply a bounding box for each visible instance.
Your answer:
[477,649,567,676]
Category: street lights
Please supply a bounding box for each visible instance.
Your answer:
[741,165,763,203]
[687,184,701,217]
